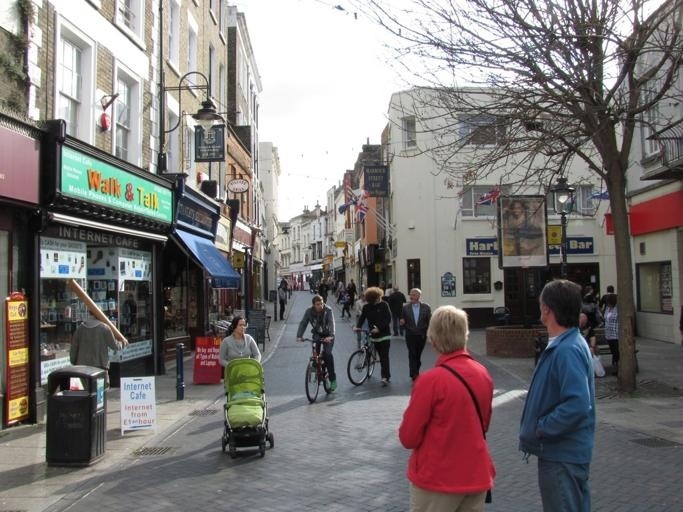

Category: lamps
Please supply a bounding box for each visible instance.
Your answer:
[162,72,223,136]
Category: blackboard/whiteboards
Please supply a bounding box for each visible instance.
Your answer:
[247,309,266,344]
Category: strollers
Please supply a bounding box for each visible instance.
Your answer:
[218,360,279,457]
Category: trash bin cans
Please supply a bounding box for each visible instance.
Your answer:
[45,365,106,467]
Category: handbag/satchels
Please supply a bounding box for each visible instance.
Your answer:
[486,491,493,503]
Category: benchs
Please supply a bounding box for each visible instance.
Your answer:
[535,333,640,380]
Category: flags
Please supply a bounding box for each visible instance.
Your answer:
[353,202,369,223]
[475,186,500,208]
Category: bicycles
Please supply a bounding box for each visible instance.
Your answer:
[298,339,338,404]
[344,331,386,384]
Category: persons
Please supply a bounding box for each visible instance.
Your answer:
[398,288,431,383]
[354,287,393,387]
[399,303,497,512]
[276,276,302,321]
[297,295,337,393]
[218,316,262,368]
[503,198,531,229]
[518,280,595,511]
[308,272,406,336]
[70,308,127,416]
[576,282,622,375]
[124,291,135,324]
[224,305,234,320]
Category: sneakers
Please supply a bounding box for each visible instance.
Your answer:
[330,380,337,391]
[381,378,387,386]
[389,376,392,382]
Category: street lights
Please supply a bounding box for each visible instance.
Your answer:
[553,173,577,285]
